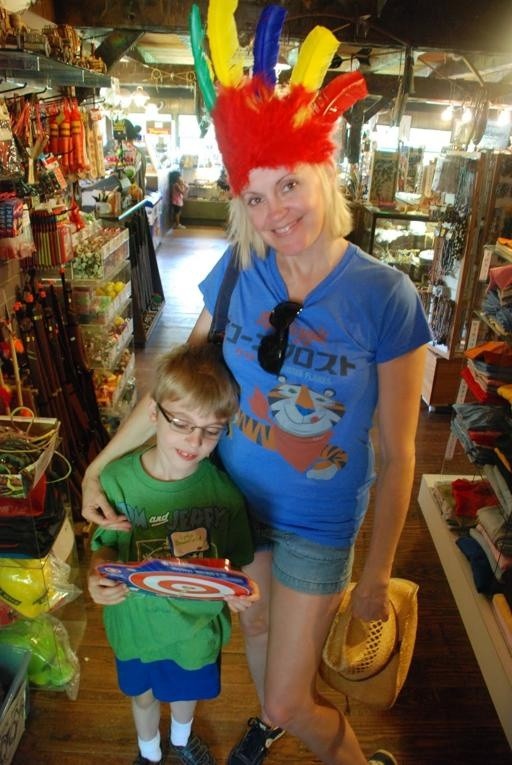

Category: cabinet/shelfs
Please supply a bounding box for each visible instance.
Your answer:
[414,242,512,753]
[358,195,440,274]
[62,230,141,433]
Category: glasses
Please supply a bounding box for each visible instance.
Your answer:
[255,299,305,378]
[156,401,231,441]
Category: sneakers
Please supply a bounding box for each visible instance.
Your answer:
[225,716,289,765]
[367,748,399,765]
[132,738,167,764]
[166,727,217,764]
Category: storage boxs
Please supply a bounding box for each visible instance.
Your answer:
[1,647,34,764]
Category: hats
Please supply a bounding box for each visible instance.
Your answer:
[315,575,422,712]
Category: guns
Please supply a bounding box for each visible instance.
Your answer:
[12,267,111,521]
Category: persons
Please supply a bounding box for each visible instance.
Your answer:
[169,171,188,232]
[111,117,165,346]
[79,156,434,765]
[84,344,257,764]
